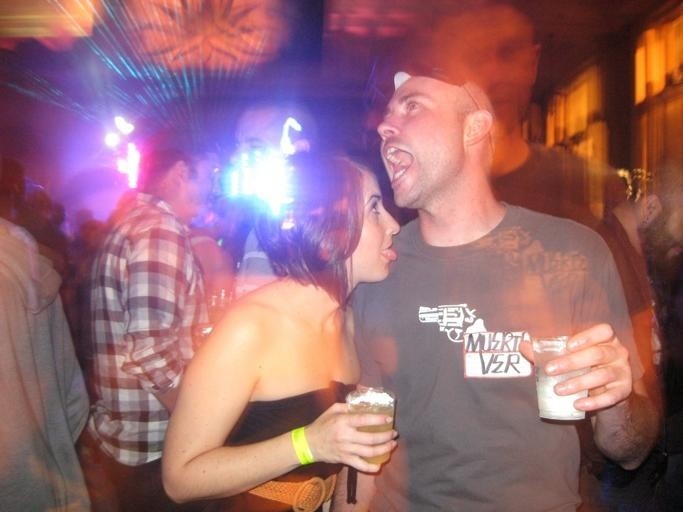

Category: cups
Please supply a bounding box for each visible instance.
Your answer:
[531,336,588,422]
[345,386,397,465]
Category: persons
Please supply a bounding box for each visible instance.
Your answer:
[0,1,682,512]
